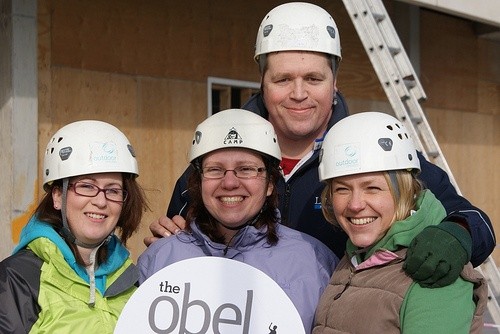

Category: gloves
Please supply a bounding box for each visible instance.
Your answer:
[402,222,471,289]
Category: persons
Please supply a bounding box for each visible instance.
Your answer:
[143,2,497,290]
[135,108,340,334]
[311,111,488,334]
[0,120,144,334]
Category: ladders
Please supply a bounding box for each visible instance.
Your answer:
[343,0,500,333]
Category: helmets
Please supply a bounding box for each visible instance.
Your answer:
[189,109,282,167]
[42,120,140,193]
[254,2,342,62]
[318,111,420,182]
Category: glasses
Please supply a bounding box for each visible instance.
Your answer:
[60,182,129,202]
[200,166,267,179]
